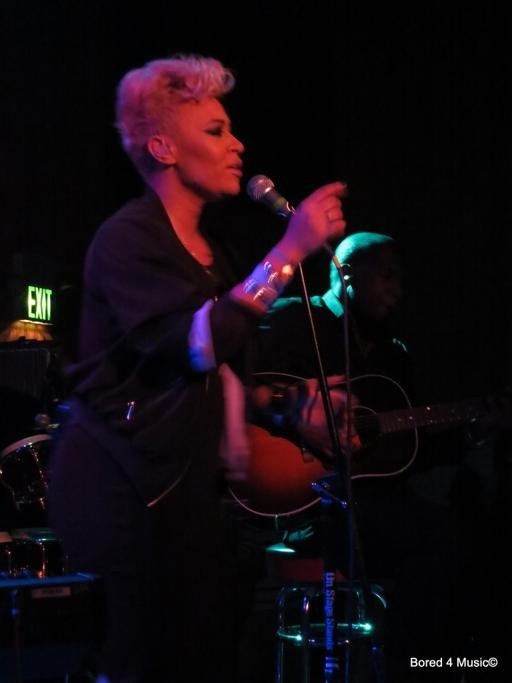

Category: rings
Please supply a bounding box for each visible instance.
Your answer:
[328,211,332,221]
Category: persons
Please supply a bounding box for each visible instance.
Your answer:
[44,59,349,677]
[232,232,403,550]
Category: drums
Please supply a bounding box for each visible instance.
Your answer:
[8,525,79,609]
[1,435,67,521]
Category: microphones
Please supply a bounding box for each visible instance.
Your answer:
[247,174,335,258]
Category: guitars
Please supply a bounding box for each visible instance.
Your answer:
[228,366,511,515]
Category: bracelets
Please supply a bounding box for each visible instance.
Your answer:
[243,260,284,309]
